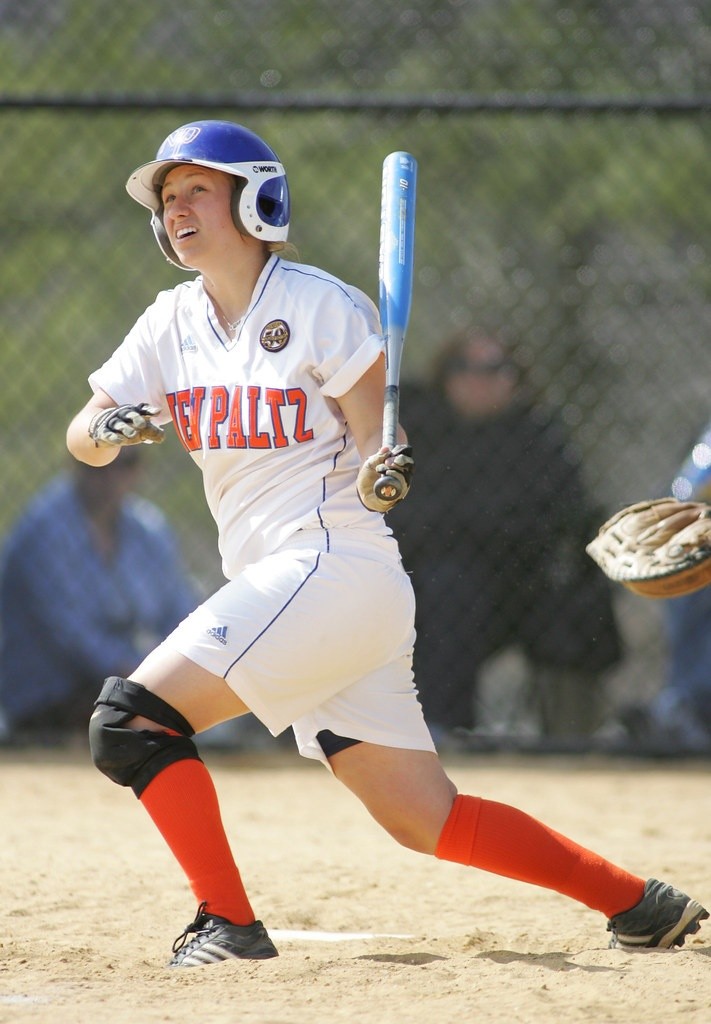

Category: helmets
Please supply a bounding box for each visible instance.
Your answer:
[124,120,291,272]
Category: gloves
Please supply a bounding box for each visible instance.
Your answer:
[88,402,166,449]
[355,442,415,513]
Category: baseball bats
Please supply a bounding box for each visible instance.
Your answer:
[373,150,420,502]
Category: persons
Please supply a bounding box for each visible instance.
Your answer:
[378,331,626,750]
[0,427,242,761]
[66,122,711,976]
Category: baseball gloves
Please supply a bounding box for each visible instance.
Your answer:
[583,495,711,599]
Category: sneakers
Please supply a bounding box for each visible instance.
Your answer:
[607,879,708,949]
[161,900,280,970]
